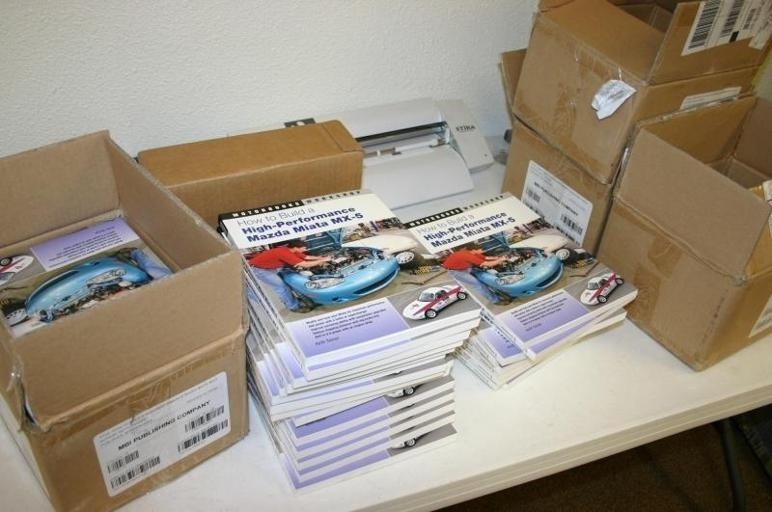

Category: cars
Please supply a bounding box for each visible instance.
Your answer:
[0,251,32,291]
[24,255,154,325]
[464,231,564,305]
[264,225,399,313]
[341,233,418,269]
[580,271,624,308]
[1,302,47,339]
[403,281,469,322]
[506,234,575,264]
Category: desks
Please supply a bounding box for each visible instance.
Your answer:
[2,135,772,512]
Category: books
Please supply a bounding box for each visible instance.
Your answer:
[403,190,640,393]
[0,218,175,338]
[214,189,483,498]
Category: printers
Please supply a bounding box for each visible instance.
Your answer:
[227,96,509,226]
